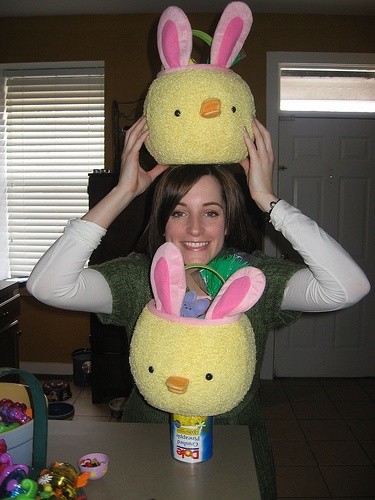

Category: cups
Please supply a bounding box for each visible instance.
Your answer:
[171,412,214,463]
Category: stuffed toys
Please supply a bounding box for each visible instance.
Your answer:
[128,241,267,418]
[142,1,256,166]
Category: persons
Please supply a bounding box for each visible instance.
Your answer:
[26,116,371,499]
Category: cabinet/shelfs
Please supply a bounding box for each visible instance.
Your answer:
[0,281,22,382]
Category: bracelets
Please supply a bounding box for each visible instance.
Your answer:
[262,198,282,222]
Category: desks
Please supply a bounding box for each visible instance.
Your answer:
[46,420,261,500]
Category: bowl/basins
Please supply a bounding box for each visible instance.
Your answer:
[48,402,75,420]
[109,397,128,417]
[78,452,109,480]
[43,380,72,402]
